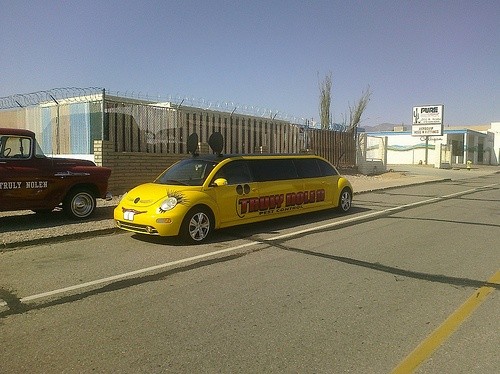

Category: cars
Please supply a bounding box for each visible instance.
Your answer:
[113,153,354,245]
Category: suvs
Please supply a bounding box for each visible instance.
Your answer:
[0,127,111,221]
